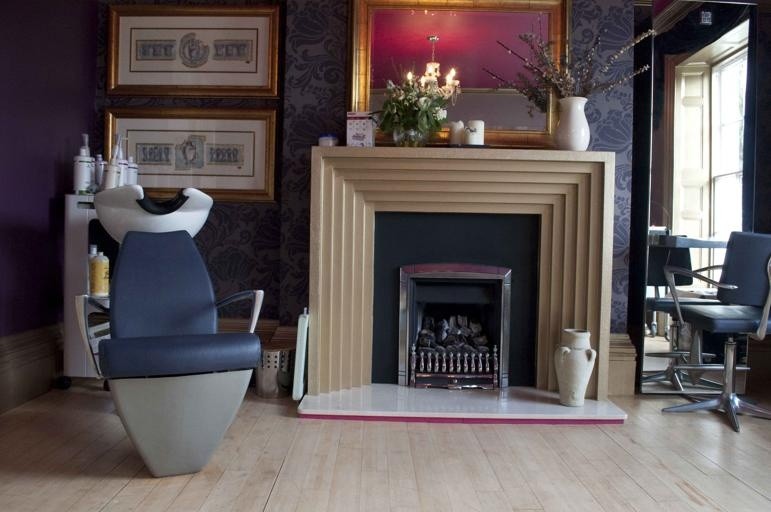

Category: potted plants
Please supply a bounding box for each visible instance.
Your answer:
[495,12,657,151]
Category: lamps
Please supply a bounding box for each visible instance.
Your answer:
[406,36,459,104]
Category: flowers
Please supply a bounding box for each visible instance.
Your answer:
[371,77,450,134]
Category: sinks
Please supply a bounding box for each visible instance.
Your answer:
[96,181,214,244]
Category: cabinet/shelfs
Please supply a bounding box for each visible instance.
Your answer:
[54,194,111,392]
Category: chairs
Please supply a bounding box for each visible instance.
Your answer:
[662,232,771,432]
[646,235,723,392]
[75,185,266,478]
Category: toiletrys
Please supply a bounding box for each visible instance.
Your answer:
[71,131,140,300]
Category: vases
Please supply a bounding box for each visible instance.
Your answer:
[392,124,428,146]
[555,329,597,407]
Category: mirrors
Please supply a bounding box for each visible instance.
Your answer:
[348,0,571,150]
[634,0,763,396]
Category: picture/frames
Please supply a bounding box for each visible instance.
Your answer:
[104,108,276,202]
[105,4,280,99]
